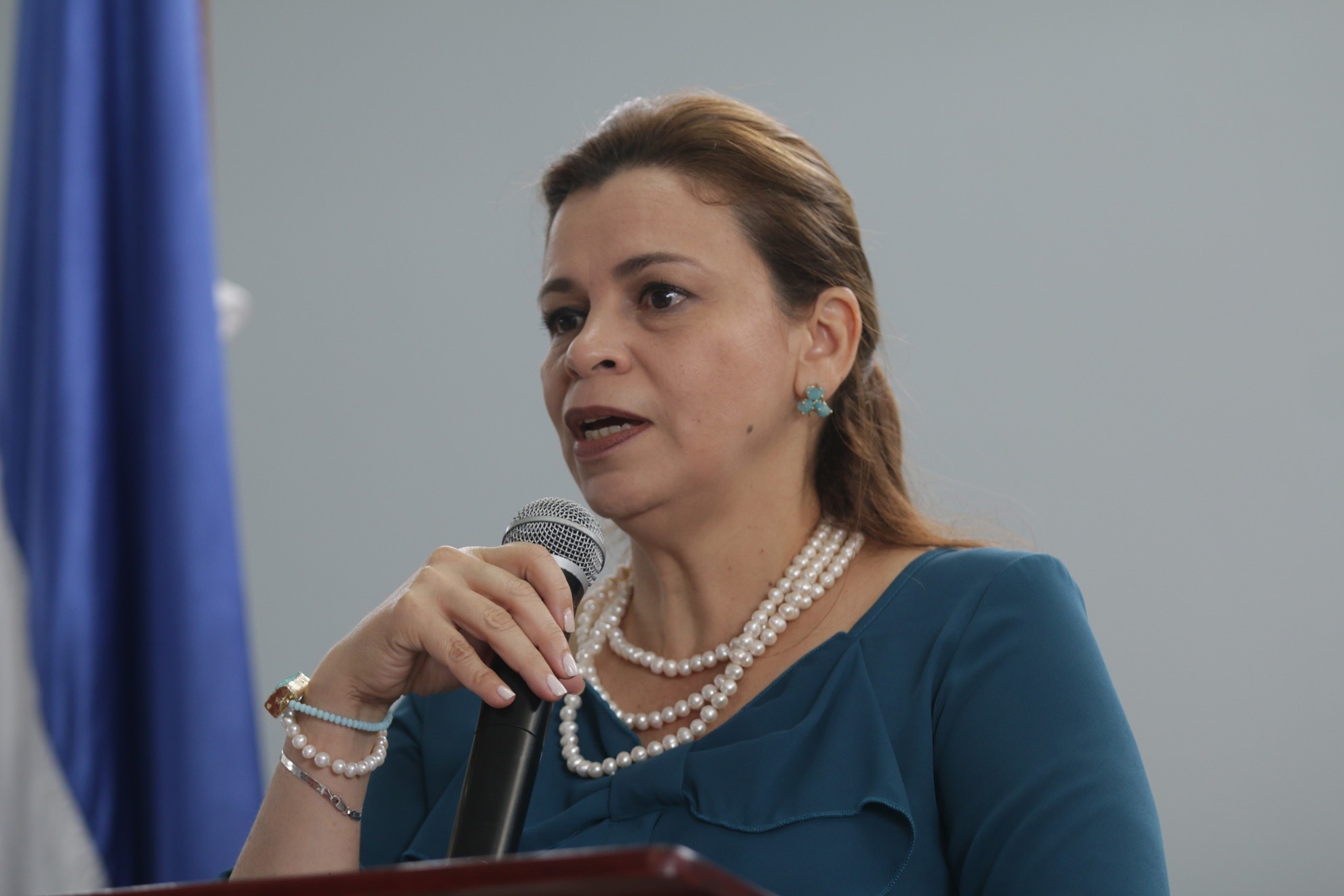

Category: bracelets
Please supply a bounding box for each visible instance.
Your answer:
[264,671,394,778]
[281,750,362,821]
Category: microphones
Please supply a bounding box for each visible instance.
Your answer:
[450,499,609,860]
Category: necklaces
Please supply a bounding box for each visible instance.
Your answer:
[557,507,866,778]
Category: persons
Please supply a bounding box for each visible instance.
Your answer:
[226,93,1173,896]
